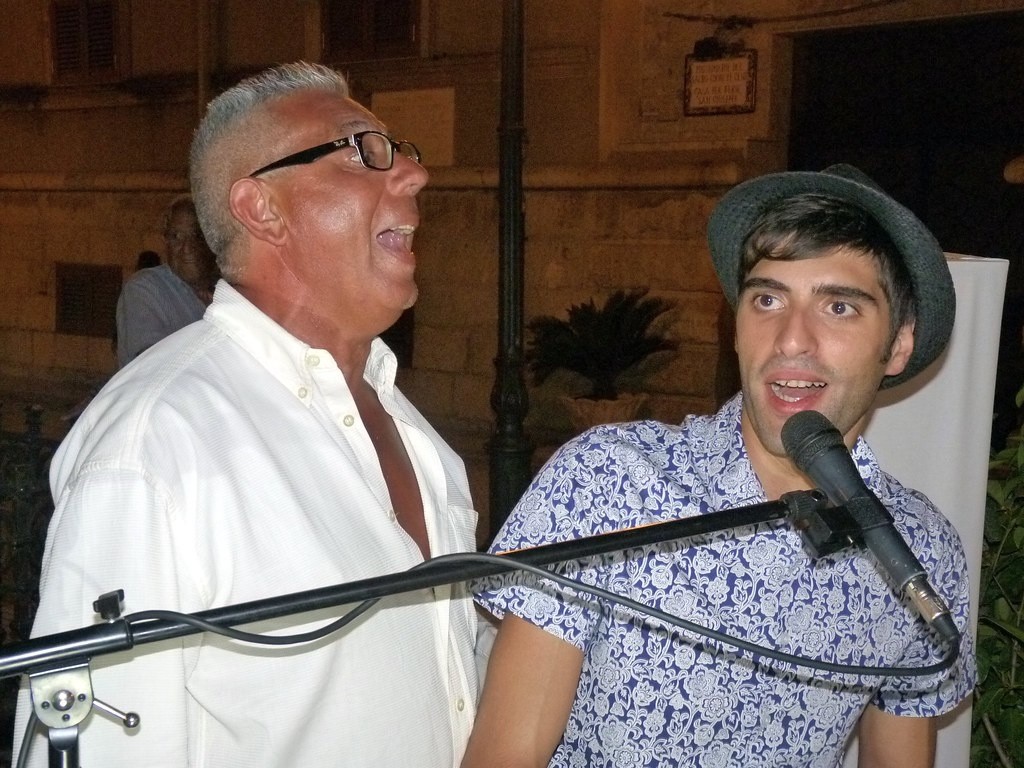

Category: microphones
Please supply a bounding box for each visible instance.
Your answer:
[779,409,962,646]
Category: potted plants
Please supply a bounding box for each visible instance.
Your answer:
[525,286,684,437]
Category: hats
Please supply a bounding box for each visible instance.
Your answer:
[707,163,956,391]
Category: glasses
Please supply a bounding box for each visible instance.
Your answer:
[249,131,423,178]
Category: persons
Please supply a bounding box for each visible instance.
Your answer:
[460,161,978,768]
[10,59,498,768]
